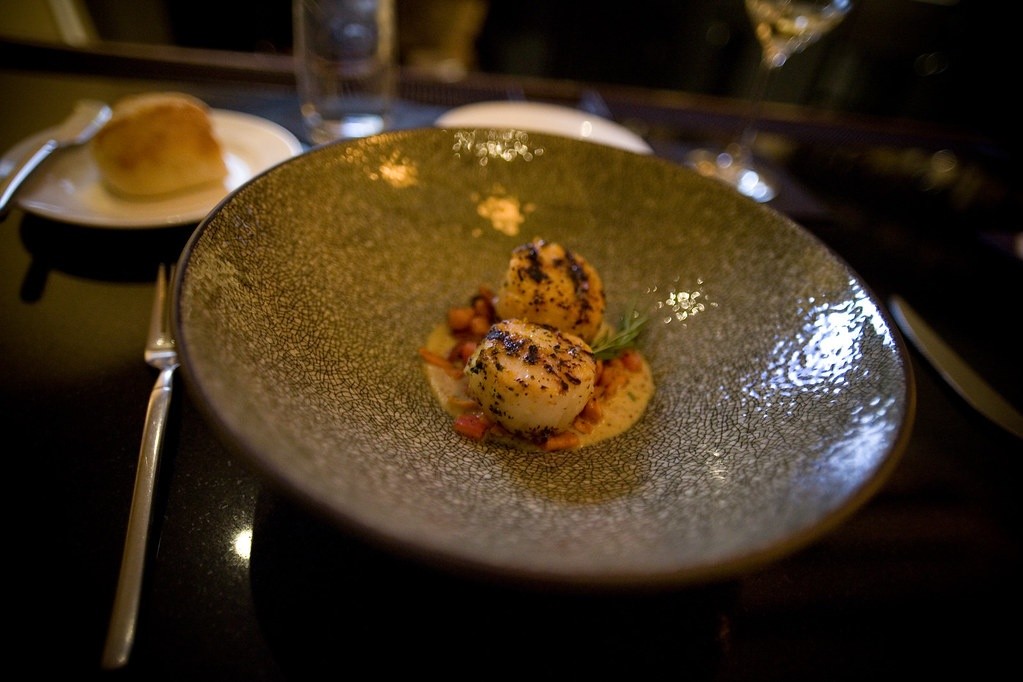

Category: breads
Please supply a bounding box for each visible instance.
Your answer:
[91,90,226,199]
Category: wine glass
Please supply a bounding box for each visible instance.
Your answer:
[685,0,854,203]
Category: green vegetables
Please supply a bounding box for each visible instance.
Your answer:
[592,294,648,360]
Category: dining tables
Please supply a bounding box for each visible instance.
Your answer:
[0,48,1023,682]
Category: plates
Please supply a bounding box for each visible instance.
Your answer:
[170,128,916,592]
[435,101,652,156]
[0,108,302,227]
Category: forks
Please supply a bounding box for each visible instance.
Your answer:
[99,265,179,668]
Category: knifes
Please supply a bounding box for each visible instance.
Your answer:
[0,98,111,209]
[895,300,1023,438]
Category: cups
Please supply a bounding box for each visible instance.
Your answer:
[294,0,394,147]
